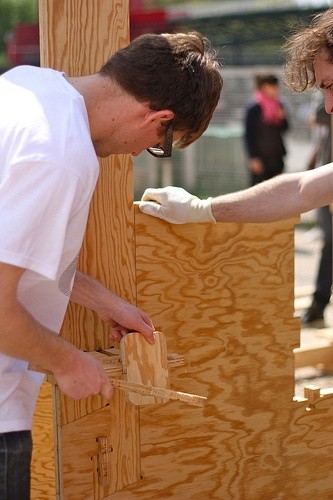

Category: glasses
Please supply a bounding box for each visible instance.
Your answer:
[147,119,174,158]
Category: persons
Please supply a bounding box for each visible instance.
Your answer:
[0,31,224,500]
[139,6,333,330]
[243,74,289,185]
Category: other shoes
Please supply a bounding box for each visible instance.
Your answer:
[301,304,325,329]
